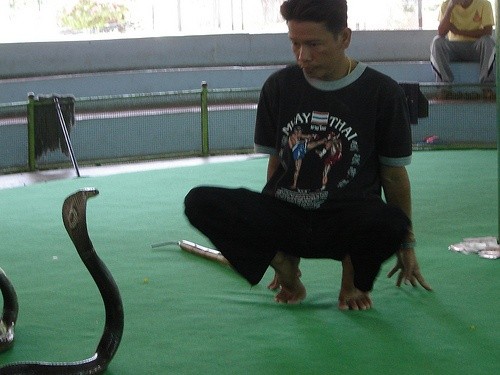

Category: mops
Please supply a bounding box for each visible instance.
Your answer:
[26,94,81,178]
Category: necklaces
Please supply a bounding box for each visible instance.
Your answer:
[345,54,352,76]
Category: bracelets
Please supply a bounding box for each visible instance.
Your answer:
[399,242,416,248]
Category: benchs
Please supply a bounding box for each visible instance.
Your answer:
[1,29,495,176]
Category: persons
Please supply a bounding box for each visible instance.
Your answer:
[183,0,431,311]
[428,0,497,101]
